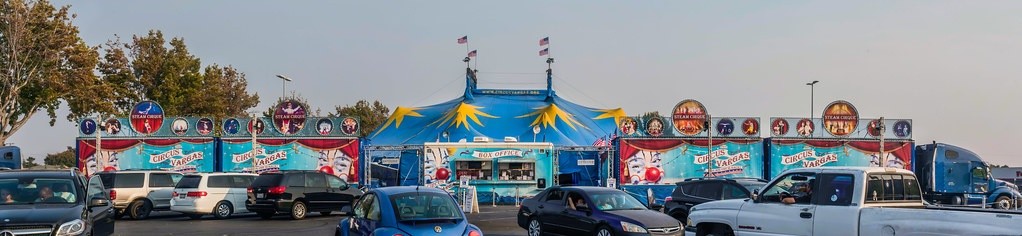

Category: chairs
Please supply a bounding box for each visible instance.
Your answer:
[52,182,67,192]
[399,205,451,217]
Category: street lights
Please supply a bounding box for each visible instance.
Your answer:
[276,74,292,101]
[805,80,819,138]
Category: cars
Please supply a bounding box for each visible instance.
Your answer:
[518,185,686,236]
[335,186,484,236]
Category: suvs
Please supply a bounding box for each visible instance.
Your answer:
[245,169,365,220]
[662,176,792,230]
[94,169,184,221]
[0,166,115,236]
[169,172,260,220]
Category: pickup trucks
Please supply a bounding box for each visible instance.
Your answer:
[686,165,1021,236]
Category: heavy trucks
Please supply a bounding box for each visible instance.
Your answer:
[612,116,1022,213]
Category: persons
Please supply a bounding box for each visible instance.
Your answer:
[568,197,612,211]
[0,187,76,204]
[781,179,815,204]
